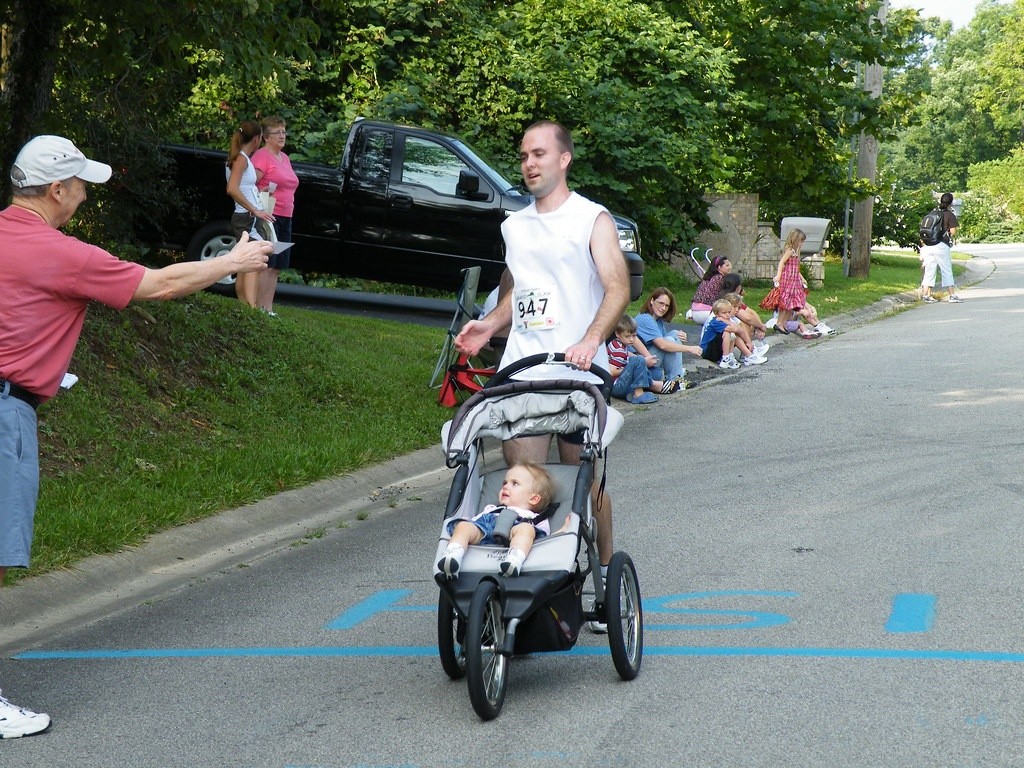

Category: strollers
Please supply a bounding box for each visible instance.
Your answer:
[689,247,745,302]
[428,349,643,721]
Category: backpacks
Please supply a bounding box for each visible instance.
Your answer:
[920,206,950,245]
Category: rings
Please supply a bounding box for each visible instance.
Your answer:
[579,357,585,361]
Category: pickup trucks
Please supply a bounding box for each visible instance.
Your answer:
[120,116,646,304]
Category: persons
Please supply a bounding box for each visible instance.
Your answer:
[629,287,703,395]
[691,256,744,324]
[700,273,769,369]
[250,117,300,311]
[921,193,963,303]
[438,458,555,578]
[607,314,660,404]
[225,120,277,309]
[0,136,274,739]
[759,227,836,339]
[452,119,631,637]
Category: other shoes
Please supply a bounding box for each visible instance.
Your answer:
[498,556,520,578]
[439,555,460,580]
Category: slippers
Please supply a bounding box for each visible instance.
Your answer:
[654,380,679,393]
[627,389,659,404]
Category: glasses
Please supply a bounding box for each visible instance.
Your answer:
[269,130,287,136]
[656,300,670,309]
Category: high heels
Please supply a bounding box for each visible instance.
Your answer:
[774,325,789,334]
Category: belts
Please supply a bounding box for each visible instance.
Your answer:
[0,380,41,410]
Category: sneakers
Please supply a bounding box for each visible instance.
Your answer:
[923,295,937,303]
[719,357,741,369]
[814,323,835,335]
[753,344,770,356]
[744,353,767,366]
[949,295,963,303]
[802,330,822,339]
[588,577,608,632]
[0,696,51,739]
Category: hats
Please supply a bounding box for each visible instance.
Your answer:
[11,135,111,188]
